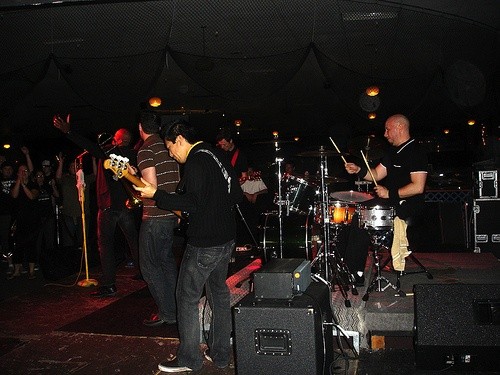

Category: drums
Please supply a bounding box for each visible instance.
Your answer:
[259,209,324,274]
[358,205,395,230]
[274,174,320,216]
[315,206,355,227]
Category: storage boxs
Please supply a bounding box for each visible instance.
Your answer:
[464,169,500,254]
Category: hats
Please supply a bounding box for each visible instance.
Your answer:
[41,160,51,166]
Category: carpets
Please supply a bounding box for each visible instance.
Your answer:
[61,263,236,343]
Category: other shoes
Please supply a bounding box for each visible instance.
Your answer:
[204,349,228,368]
[158,356,200,373]
[88,284,118,297]
[34,264,40,270]
[130,272,145,280]
[143,313,173,327]
[6,265,14,274]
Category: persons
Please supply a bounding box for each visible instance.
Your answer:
[334,115,429,286]
[88,128,139,298]
[133,121,242,372]
[126,110,181,325]
[0,147,98,280]
[217,130,300,247]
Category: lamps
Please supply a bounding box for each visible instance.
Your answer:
[147,95,164,108]
[367,84,381,102]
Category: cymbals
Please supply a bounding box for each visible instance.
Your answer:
[330,191,374,203]
[325,175,348,183]
[297,150,349,157]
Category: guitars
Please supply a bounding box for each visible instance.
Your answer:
[239,171,262,183]
[103,155,190,234]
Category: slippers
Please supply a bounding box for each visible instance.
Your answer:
[29,273,36,280]
[7,272,23,280]
